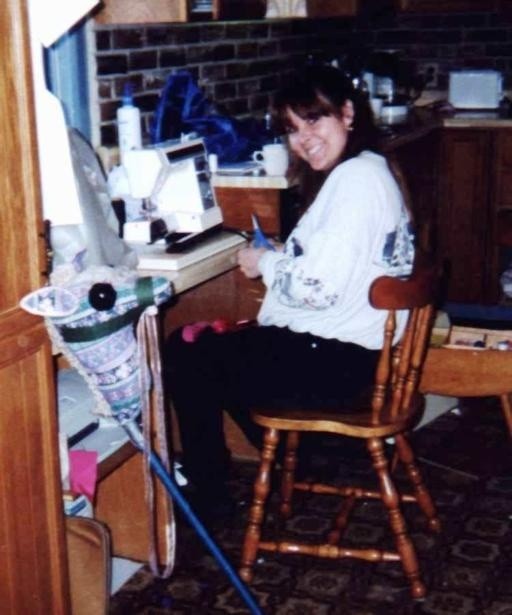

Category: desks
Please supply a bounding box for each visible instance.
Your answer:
[131,242,250,294]
[388,344,512,476]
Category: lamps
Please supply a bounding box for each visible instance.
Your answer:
[264,0,309,20]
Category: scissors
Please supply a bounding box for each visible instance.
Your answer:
[251,212,276,251]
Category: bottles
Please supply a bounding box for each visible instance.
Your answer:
[116,82,144,162]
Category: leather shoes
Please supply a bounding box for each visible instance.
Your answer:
[175,483,236,514]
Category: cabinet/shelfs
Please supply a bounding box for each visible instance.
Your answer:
[97,0,357,28]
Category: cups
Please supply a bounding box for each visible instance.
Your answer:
[369,99,382,118]
[254,143,289,178]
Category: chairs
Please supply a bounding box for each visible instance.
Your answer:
[240,268,449,601]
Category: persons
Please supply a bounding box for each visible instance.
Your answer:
[165,66,421,528]
